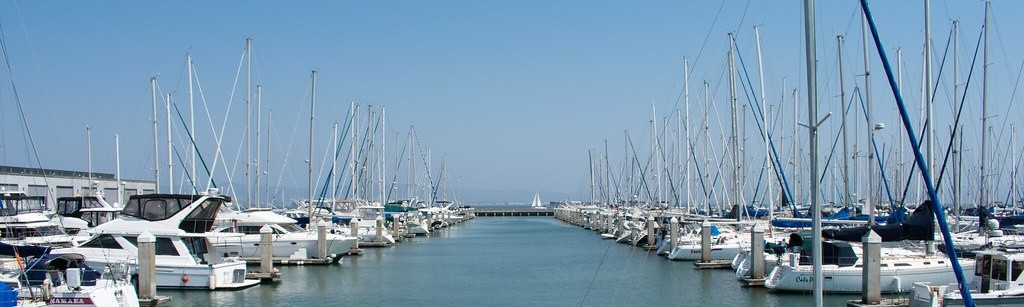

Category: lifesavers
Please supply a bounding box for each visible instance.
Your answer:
[42,279,52,301]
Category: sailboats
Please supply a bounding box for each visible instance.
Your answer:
[0,17,477,307]
[528,0,1024,307]
